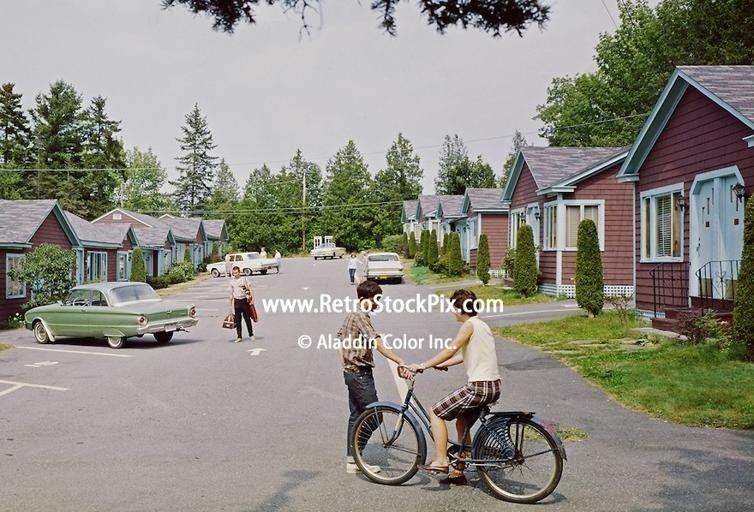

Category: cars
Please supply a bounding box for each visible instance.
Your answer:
[22,280,199,350]
[364,249,405,285]
[203,251,280,278]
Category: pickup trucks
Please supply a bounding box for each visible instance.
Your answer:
[309,243,347,261]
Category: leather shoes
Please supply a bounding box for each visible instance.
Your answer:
[440,475,466,485]
[417,462,449,476]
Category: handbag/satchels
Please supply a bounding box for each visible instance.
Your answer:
[223,313,236,329]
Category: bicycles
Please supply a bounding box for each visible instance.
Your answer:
[347,360,567,505]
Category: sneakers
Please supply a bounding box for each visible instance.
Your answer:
[345,462,380,474]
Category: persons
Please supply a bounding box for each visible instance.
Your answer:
[259,247,267,259]
[274,249,282,274]
[408,289,503,485]
[229,266,256,343]
[335,280,413,474]
[346,250,367,285]
